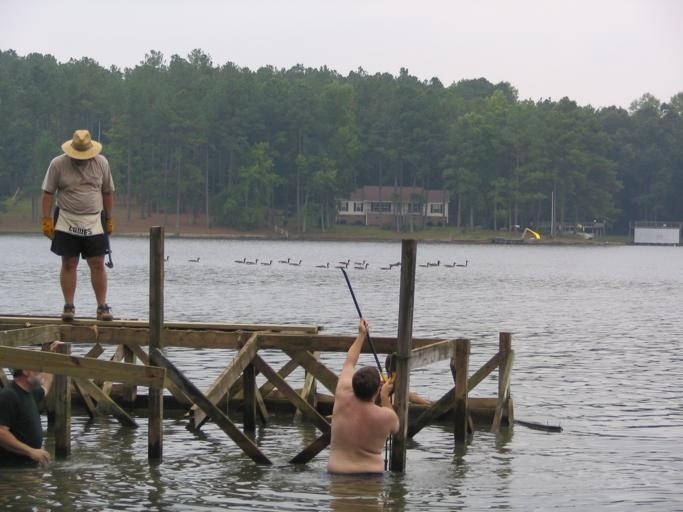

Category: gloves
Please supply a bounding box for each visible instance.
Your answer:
[40,217,54,239]
[105,217,113,235]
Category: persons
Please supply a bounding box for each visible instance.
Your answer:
[0,340,68,467]
[37,128,117,322]
[373,351,432,407]
[324,319,403,480]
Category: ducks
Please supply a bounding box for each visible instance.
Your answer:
[335,259,370,270]
[418,260,468,268]
[315,263,330,268]
[164,255,169,261]
[234,258,302,266]
[188,257,200,262]
[381,262,401,270]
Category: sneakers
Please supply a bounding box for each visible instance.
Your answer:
[62,304,75,322]
[97,307,113,321]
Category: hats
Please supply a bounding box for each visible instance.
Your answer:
[61,129,102,160]
